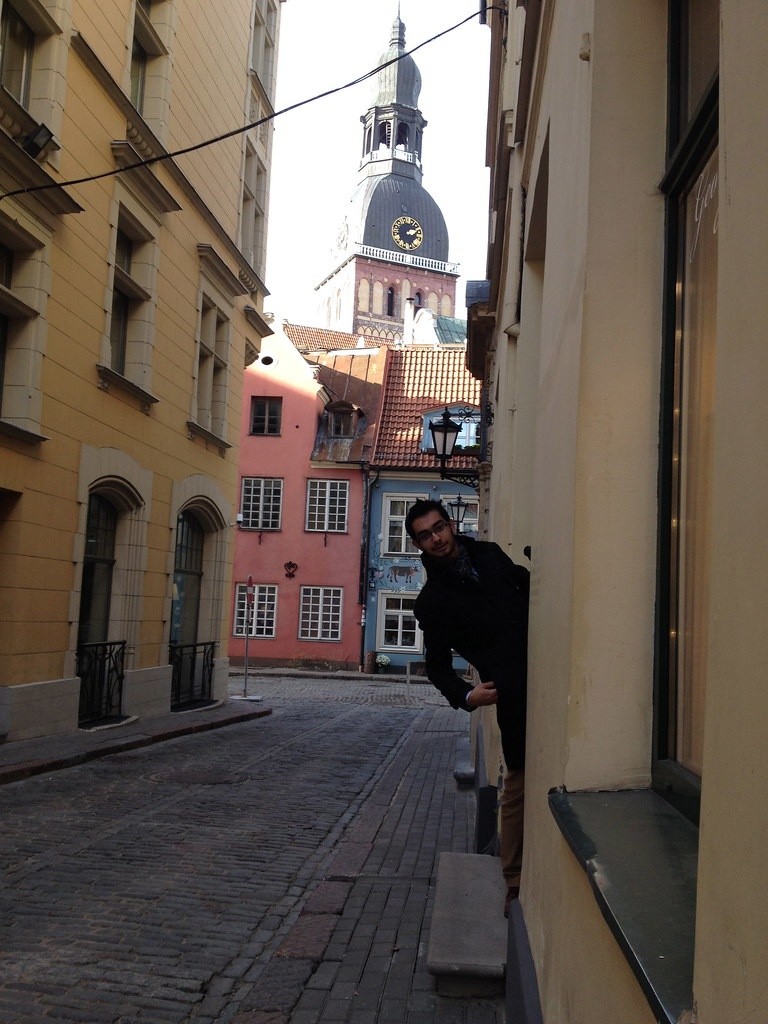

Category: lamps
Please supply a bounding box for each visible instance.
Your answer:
[447,492,478,535]
[429,400,494,491]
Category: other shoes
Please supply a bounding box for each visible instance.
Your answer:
[503,887,519,918]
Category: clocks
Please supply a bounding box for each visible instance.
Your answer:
[392,216,424,250]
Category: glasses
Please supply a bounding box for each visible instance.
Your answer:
[417,521,448,542]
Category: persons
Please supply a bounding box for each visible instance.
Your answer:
[404,499,533,917]
